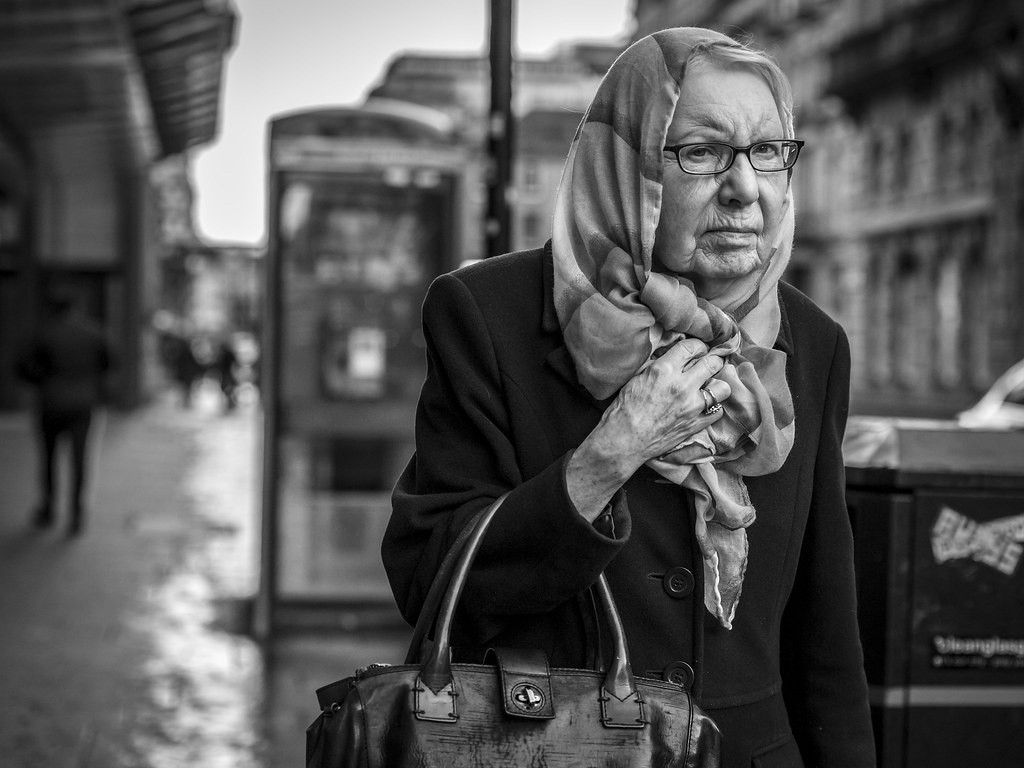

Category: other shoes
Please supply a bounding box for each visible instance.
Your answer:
[31,507,52,529]
[66,506,84,538]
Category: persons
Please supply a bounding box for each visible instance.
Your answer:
[381,27,877,768]
[174,338,239,410]
[14,268,110,535]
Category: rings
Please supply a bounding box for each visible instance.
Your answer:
[700,387,722,415]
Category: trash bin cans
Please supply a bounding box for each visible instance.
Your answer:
[843,416,1024,767]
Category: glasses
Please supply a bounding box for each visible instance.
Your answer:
[663,140,804,175]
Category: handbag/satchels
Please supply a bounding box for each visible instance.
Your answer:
[305,489,724,768]
